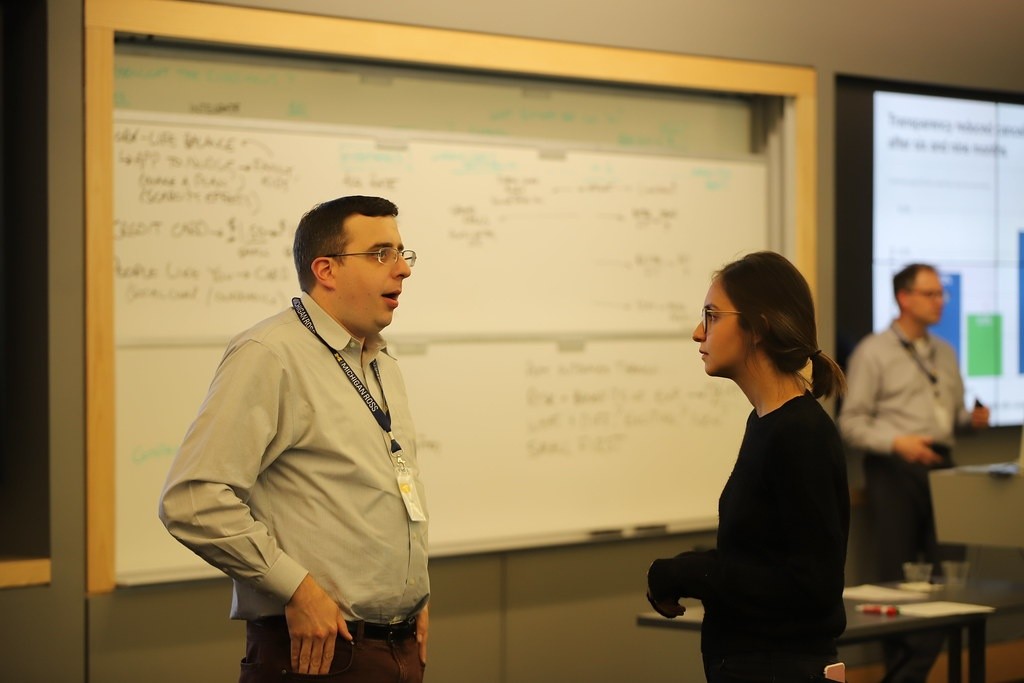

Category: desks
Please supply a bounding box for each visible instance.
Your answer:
[634,578,1000,683]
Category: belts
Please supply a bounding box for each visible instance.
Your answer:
[248,615,416,644]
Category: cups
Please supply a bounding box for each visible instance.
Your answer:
[903,552,934,586]
[940,559,971,590]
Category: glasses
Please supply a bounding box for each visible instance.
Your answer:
[701,307,742,335]
[325,247,418,267]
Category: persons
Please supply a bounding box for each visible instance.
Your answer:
[647,250,846,683]
[158,197,431,683]
[839,263,990,682]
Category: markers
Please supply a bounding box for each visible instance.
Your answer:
[853,605,896,617]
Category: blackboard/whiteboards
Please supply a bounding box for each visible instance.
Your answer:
[104,110,766,584]
